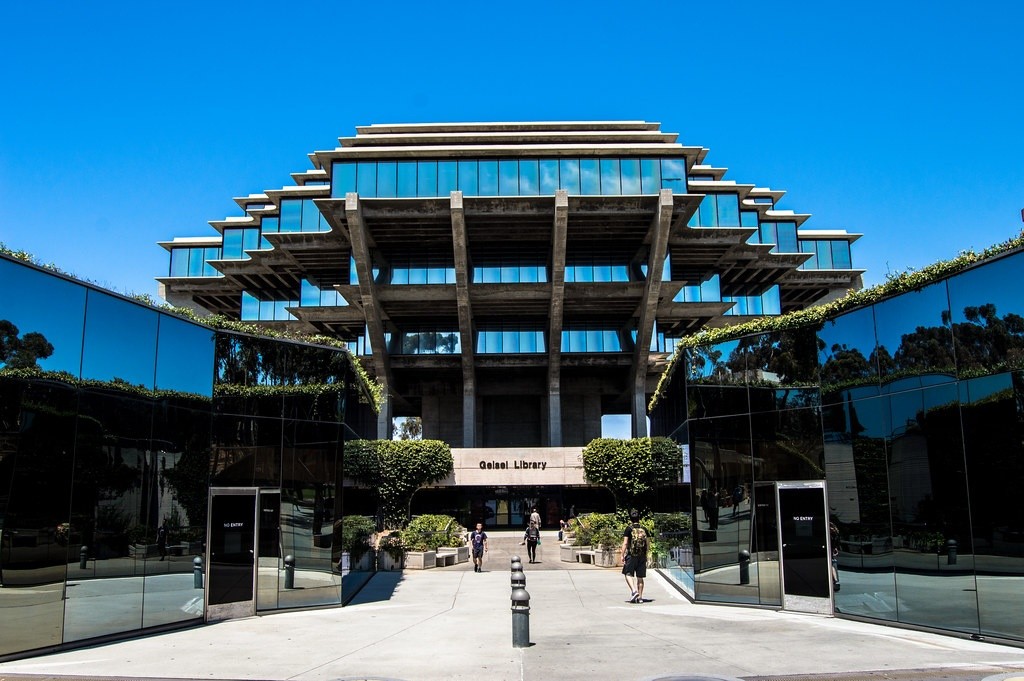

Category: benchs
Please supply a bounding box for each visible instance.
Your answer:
[575,549,597,565]
[436,552,458,567]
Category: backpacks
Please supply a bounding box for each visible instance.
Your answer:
[626,524,648,558]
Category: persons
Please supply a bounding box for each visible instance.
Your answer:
[699,483,742,523]
[520,519,541,562]
[830,522,841,591]
[292,492,300,511]
[558,519,570,539]
[156,518,169,561]
[622,511,651,604]
[570,505,575,518]
[471,523,488,572]
[530,509,541,540]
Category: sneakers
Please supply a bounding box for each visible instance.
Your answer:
[629,593,645,603]
